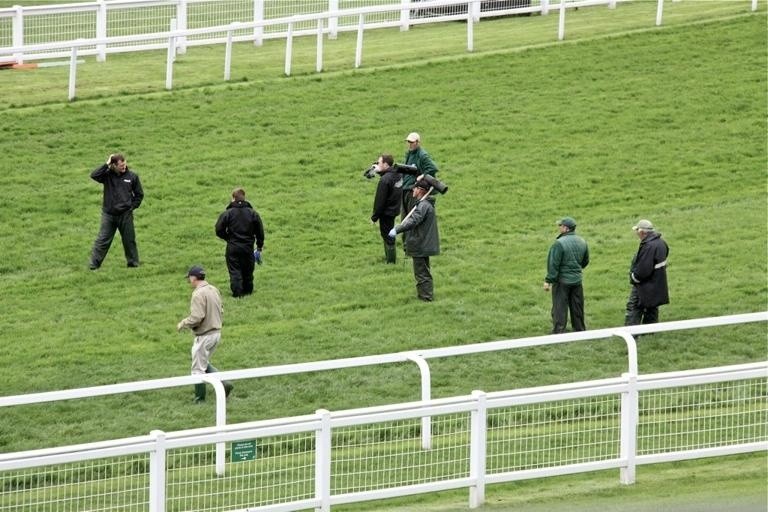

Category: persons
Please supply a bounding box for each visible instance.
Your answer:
[178,266,234,403]
[388,179,440,301]
[544,218,589,331]
[88,155,144,271]
[623,220,669,338]
[214,190,264,297]
[369,155,402,264]
[402,133,437,216]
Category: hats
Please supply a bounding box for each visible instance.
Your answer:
[556,217,577,228]
[632,219,655,233]
[404,132,420,142]
[184,265,206,279]
[408,181,429,190]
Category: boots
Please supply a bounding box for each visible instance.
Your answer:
[195,383,206,402]
[205,362,234,398]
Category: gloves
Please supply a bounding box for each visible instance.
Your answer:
[388,228,396,237]
[253,249,262,265]
[176,320,186,334]
